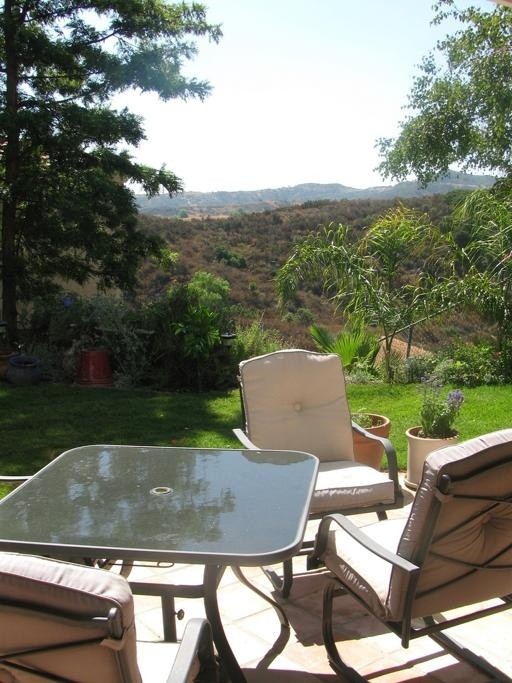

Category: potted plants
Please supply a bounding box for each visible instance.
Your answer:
[349,411,390,472]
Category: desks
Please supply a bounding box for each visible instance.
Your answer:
[0,446,321,682]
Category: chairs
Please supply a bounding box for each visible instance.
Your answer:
[232,347,408,600]
[0,548,220,683]
[305,429,512,679]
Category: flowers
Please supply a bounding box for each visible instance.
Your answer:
[414,371,465,438]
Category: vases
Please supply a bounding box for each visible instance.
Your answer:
[7,354,42,385]
[403,426,461,490]
[1,348,18,380]
